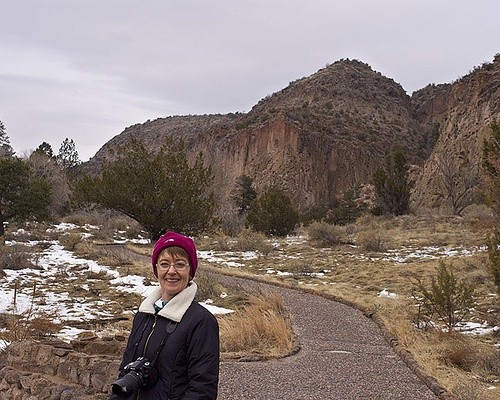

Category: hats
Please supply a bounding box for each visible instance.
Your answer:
[152,230,198,281]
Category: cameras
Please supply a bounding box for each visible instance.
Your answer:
[111,357,158,400]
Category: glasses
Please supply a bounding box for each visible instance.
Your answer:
[156,263,189,270]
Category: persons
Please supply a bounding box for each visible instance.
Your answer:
[109,231,220,400]
